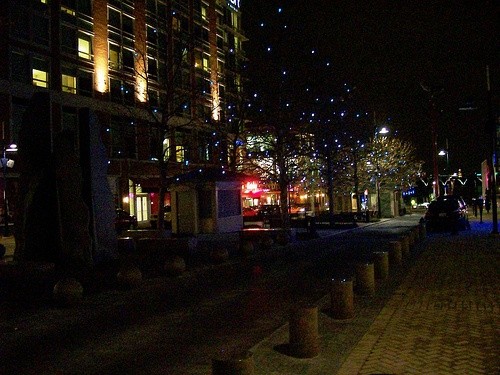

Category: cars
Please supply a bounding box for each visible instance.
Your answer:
[148,205,173,228]
[115,208,138,232]
[424,197,467,231]
[444,194,470,215]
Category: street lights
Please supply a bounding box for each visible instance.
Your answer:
[438,149,449,179]
[374,124,390,220]
